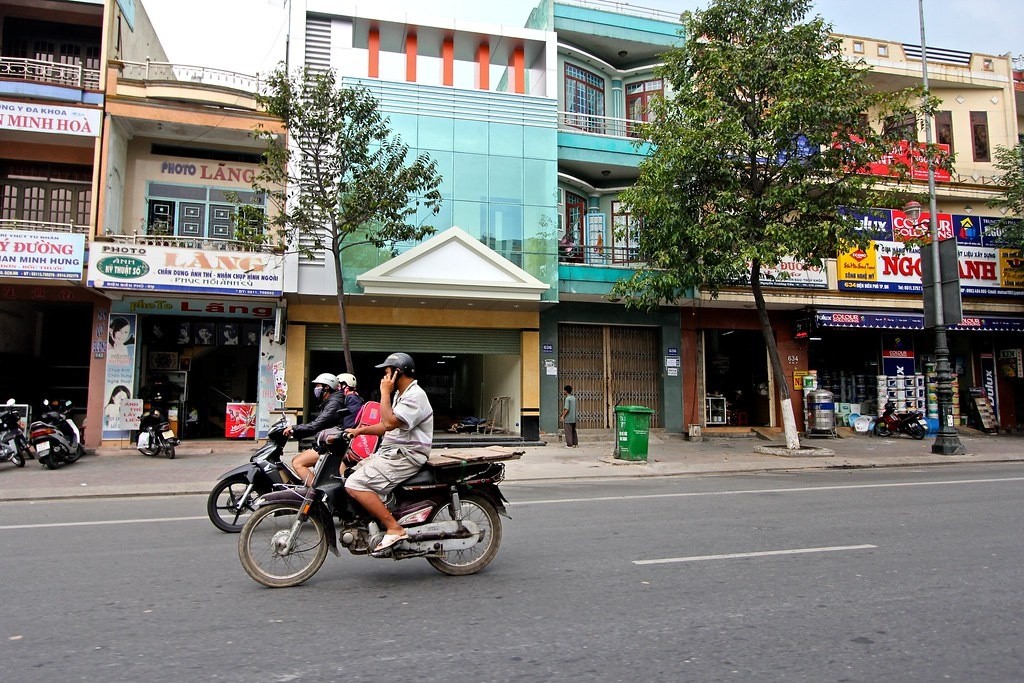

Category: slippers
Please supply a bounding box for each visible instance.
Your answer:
[373,534,409,551]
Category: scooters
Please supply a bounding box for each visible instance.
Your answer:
[30,399,83,470]
[0,399,34,467]
[135,408,182,459]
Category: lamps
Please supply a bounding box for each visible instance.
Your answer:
[618,51,627,57]
[601,171,611,176]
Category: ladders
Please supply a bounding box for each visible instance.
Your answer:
[483,396,510,435]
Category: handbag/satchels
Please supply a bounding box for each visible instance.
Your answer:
[137,432,150,449]
[345,401,383,464]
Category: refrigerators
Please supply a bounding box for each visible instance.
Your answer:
[225,402,256,438]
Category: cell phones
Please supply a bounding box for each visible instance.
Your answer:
[391,370,401,384]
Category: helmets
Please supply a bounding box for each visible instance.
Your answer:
[336,372,357,387]
[311,373,339,390]
[374,353,415,377]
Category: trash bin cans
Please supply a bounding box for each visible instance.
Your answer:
[615,405,656,461]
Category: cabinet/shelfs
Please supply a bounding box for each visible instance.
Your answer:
[146,370,189,439]
[168,420,181,438]
[0,404,33,442]
[706,397,727,427]
[53,365,90,410]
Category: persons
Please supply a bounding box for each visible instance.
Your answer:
[283,373,345,486]
[103,385,131,427]
[344,353,434,551]
[337,374,364,477]
[109,317,131,355]
[186,406,198,426]
[561,385,578,448]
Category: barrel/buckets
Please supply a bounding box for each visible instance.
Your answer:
[803,363,968,431]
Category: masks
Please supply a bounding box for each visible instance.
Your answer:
[314,386,327,398]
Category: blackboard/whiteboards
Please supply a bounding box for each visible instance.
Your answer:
[120,399,143,429]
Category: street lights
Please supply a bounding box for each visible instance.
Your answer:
[904,201,968,456]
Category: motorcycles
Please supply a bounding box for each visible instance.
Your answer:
[207,413,362,533]
[238,426,512,588]
[874,395,928,440]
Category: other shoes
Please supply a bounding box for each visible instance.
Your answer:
[564,445,580,449]
